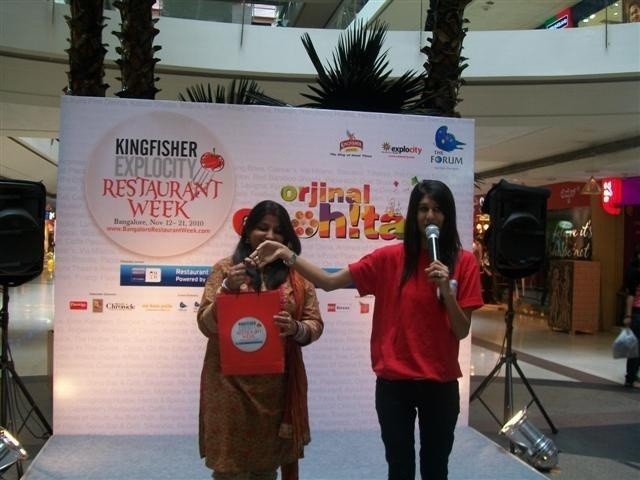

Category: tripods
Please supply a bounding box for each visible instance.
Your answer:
[0,287,54,439]
[469,279,557,454]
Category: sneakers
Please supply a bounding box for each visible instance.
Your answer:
[624,373,639,389]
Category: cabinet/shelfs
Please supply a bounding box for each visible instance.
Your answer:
[547,258,601,334]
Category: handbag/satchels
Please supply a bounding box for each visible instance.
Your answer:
[214,256,287,377]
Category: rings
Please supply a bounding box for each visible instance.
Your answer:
[438,271,441,277]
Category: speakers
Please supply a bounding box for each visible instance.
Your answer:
[0,180,46,287]
[490,183,548,279]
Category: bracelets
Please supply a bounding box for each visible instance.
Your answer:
[222,277,233,293]
[625,314,632,319]
[282,252,297,267]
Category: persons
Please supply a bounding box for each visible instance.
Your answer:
[481,250,497,305]
[245,180,484,480]
[613,273,640,388]
[197,199,324,480]
[472,234,484,267]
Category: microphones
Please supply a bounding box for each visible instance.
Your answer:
[424,224,441,280]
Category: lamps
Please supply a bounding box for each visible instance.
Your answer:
[497,406,560,471]
[0,427,29,477]
